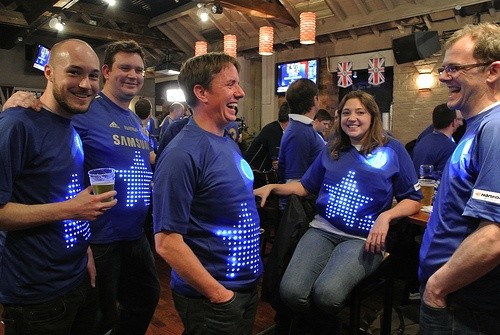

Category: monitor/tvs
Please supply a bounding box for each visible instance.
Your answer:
[32,43,51,74]
[275,57,320,96]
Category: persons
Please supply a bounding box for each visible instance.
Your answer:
[254,91,424,335]
[135,97,239,176]
[2,41,160,335]
[0,38,117,335]
[420,22,500,335]
[149,52,263,335]
[404,105,467,180]
[242,78,331,257]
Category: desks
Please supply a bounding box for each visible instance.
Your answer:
[378,211,431,335]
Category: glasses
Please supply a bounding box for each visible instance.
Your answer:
[438,61,496,75]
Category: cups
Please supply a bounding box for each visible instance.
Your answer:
[88,168,115,202]
[420,165,435,179]
[418,179,435,206]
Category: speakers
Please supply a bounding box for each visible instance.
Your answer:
[0,24,20,49]
[392,31,441,64]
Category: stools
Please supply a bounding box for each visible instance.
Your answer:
[345,252,394,335]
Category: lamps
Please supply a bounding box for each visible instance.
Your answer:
[223,8,238,57]
[258,3,274,56]
[415,72,434,92]
[211,0,223,14]
[194,22,207,56]
[154,48,186,76]
[300,0,317,45]
